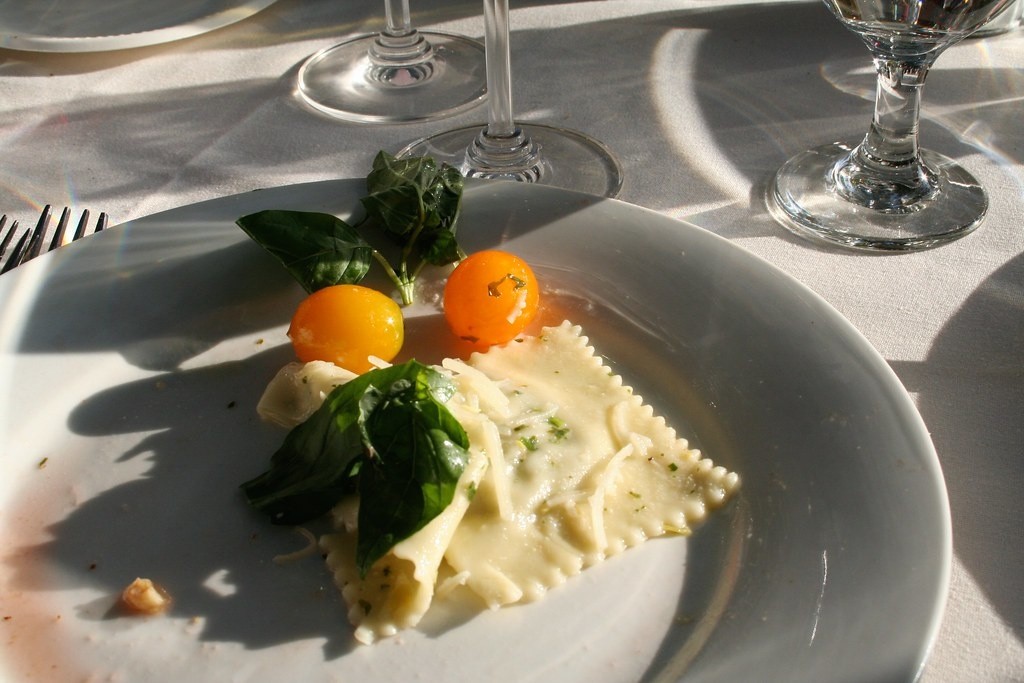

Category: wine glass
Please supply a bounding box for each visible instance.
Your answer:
[298,0,488,124]
[393,0,623,200]
[765,0,1024,252]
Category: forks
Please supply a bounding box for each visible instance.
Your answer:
[0,202,108,276]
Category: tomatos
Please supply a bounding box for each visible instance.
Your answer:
[443,248,539,345]
[286,283,404,375]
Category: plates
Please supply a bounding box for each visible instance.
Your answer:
[0,176,954,683]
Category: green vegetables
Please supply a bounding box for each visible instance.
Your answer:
[234,148,470,579]
